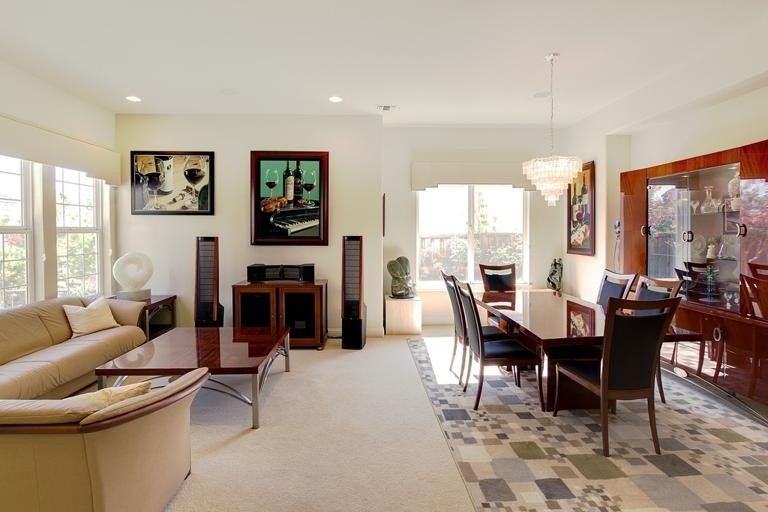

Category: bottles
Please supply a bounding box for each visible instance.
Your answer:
[155,156,173,195]
[293,160,303,201]
[283,161,294,204]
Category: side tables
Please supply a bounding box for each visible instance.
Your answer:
[109,295,177,342]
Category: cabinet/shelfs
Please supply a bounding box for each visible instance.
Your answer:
[618,139,768,426]
[232,279,328,351]
[385,293,422,335]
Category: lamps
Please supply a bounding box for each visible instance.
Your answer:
[523,52,583,207]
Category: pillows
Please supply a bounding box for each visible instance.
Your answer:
[63,296,120,339]
[63,381,151,405]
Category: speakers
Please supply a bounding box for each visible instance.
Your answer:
[343,235,367,349]
[194,236,224,326]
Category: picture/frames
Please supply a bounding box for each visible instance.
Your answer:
[130,150,216,216]
[566,160,596,256]
[250,149,328,246]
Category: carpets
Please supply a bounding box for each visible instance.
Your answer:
[406,335,768,512]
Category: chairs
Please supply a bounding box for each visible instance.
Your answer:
[631,274,685,404]
[553,297,688,457]
[454,277,546,411]
[478,263,519,336]
[596,268,638,312]
[440,270,517,386]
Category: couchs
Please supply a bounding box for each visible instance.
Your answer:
[0,368,212,512]
[0,296,147,400]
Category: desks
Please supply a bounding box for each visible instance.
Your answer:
[470,289,703,413]
